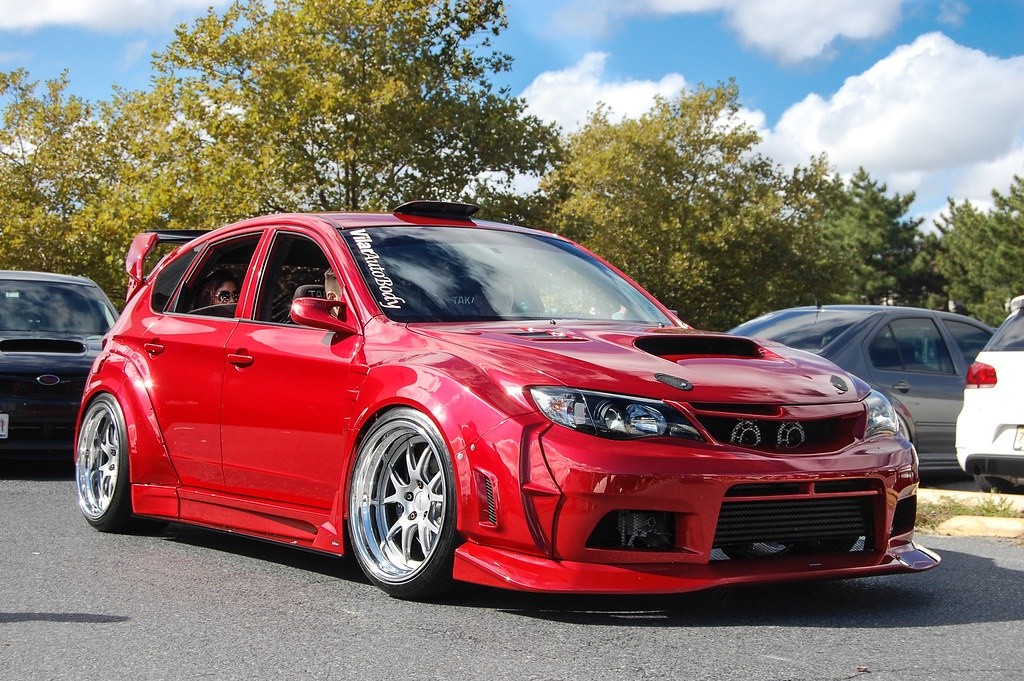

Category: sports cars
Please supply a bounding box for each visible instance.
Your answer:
[72,200,942,602]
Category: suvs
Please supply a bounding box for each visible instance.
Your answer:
[954,293,1024,495]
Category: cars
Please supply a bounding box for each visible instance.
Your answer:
[0,270,121,474]
[722,304,999,483]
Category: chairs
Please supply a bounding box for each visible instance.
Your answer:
[286,285,326,327]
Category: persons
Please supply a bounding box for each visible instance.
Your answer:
[323,267,343,318]
[195,268,240,316]
[272,260,323,325]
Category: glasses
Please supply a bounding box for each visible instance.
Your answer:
[215,289,240,303]
